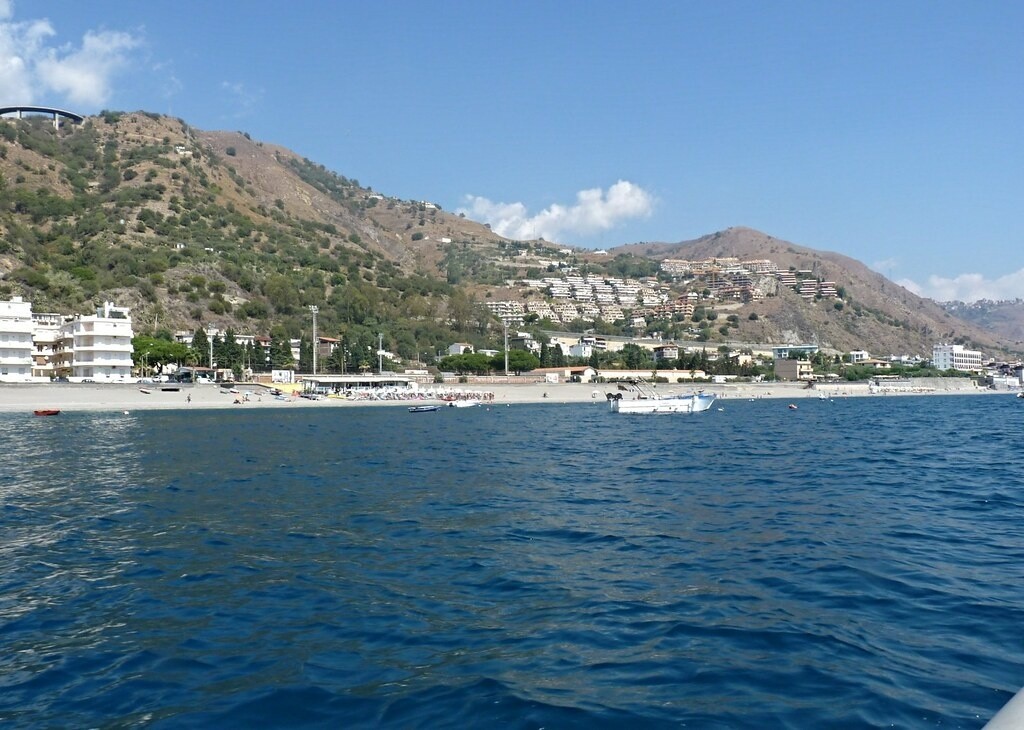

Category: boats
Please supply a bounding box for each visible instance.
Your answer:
[34,409,59,415]
[603,384,717,414]
[407,404,441,412]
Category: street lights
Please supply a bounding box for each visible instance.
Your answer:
[309,305,319,374]
[504,321,510,376]
